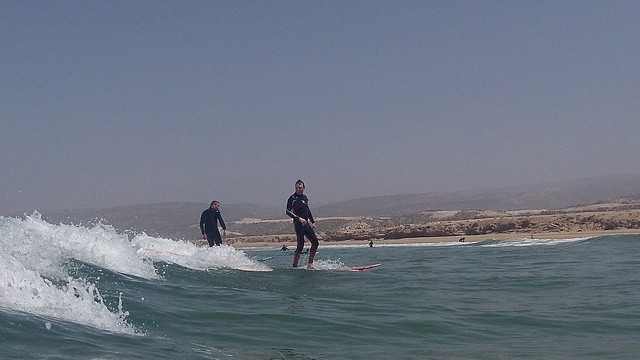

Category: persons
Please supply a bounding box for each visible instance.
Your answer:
[286,178,319,272]
[369,240,375,247]
[200,201,227,247]
[280,244,288,251]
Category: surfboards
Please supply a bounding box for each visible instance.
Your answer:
[255,257,273,261]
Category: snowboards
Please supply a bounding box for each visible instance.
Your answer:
[352,263,380,270]
